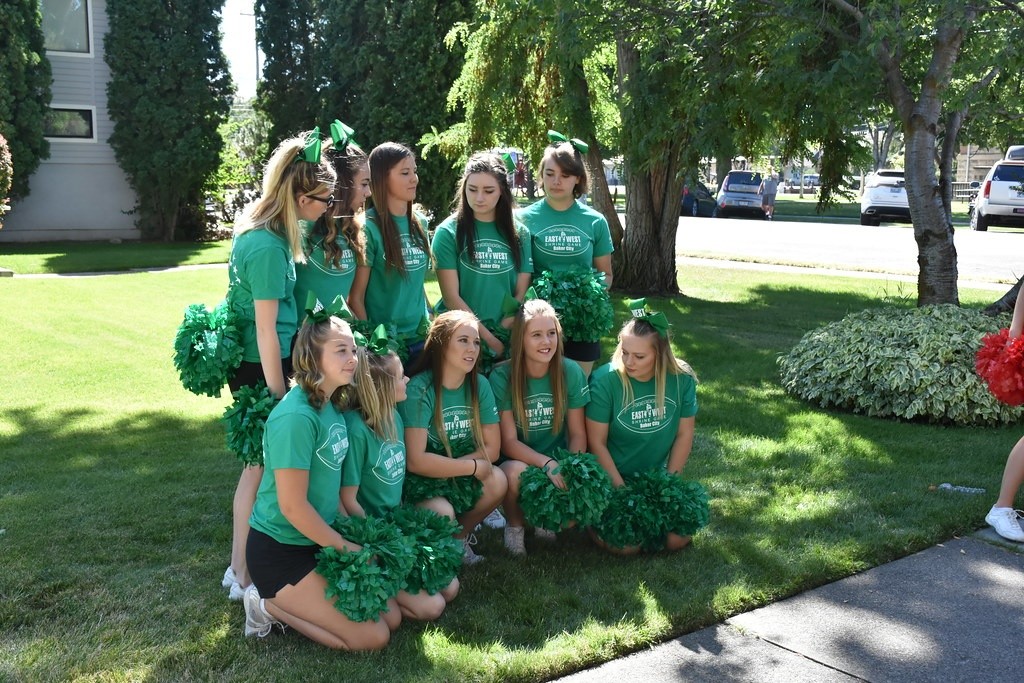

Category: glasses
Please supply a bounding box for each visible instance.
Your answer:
[299,191,334,207]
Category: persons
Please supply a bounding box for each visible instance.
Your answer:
[431,149,533,362]
[293,119,372,330]
[487,286,591,555]
[340,323,459,620]
[584,297,698,553]
[396,309,507,566]
[222,125,337,602]
[244,291,400,649]
[758,165,779,221]
[519,129,615,378]
[984,282,1024,542]
[347,142,436,359]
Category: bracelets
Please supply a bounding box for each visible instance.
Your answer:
[472,459,477,476]
[544,458,558,466]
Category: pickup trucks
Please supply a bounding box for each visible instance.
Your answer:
[844,170,876,190]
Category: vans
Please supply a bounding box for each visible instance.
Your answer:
[716,168,774,219]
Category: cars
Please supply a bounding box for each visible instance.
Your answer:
[788,173,824,187]
[679,180,717,218]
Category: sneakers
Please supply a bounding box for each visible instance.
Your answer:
[244,582,289,639]
[459,534,486,565]
[229,579,248,600]
[222,565,236,587]
[505,524,528,556]
[984,503,1024,542]
[482,511,506,528]
[537,526,558,541]
[476,524,483,532]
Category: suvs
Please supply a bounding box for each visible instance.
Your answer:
[859,168,913,226]
[967,159,1024,232]
